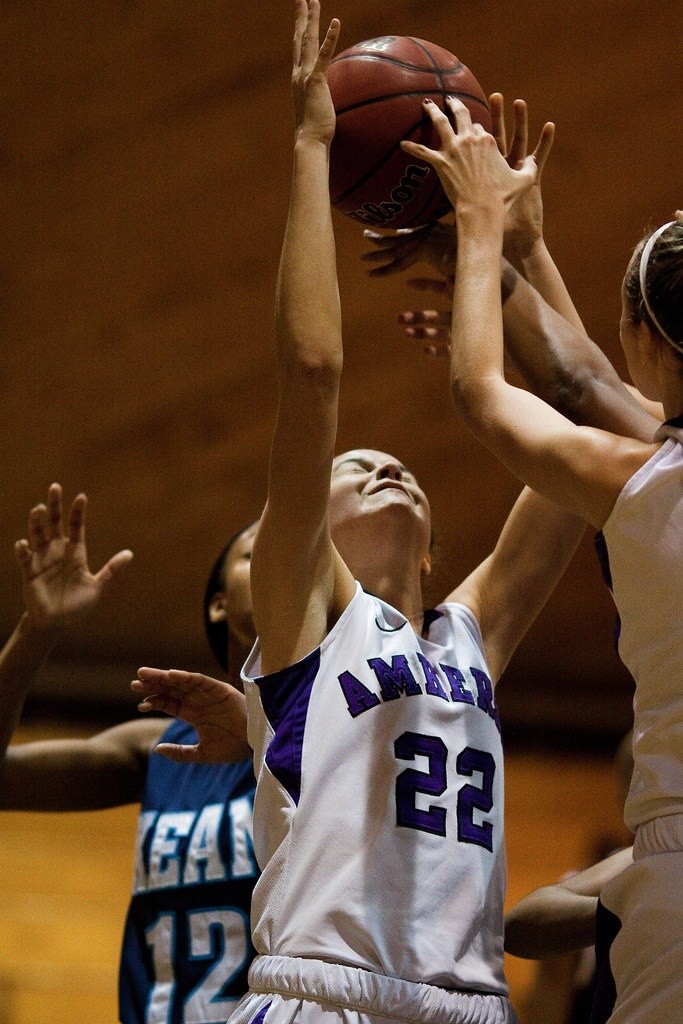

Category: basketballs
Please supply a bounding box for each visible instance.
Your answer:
[327,35,494,229]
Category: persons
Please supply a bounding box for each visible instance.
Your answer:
[225,1,589,1023]
[360,90,682,1024]
[0,482,259,1023]
